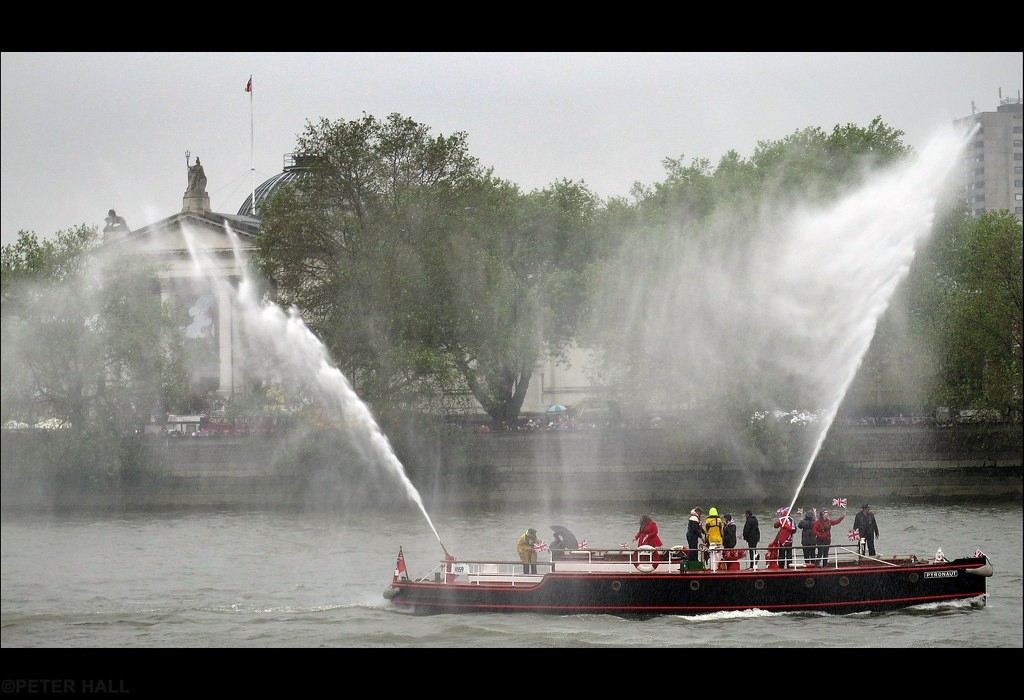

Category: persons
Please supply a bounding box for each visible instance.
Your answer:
[797,510,817,564]
[686,506,706,561]
[774,509,797,568]
[704,507,723,565]
[743,510,760,571]
[517,528,541,574]
[186,157,207,192]
[811,509,845,567]
[548,534,564,571]
[722,514,736,558]
[633,514,663,547]
[853,503,879,556]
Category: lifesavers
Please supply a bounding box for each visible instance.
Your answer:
[631,545,660,572]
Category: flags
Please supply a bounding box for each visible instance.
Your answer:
[396,549,405,573]
[245,76,252,92]
[847,528,860,542]
[832,497,847,508]
[798,509,803,514]
[578,539,588,549]
[619,543,629,550]
[775,508,784,516]
[973,550,984,558]
[812,508,816,517]
[533,541,549,552]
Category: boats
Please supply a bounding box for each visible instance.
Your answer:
[383,544,995,619]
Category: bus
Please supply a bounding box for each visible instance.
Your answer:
[199,412,275,432]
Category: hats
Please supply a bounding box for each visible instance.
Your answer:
[805,512,814,517]
[782,509,789,516]
[860,505,870,509]
[695,506,704,512]
[724,514,731,521]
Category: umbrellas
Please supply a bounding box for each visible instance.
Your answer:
[550,525,578,550]
[548,405,567,412]
